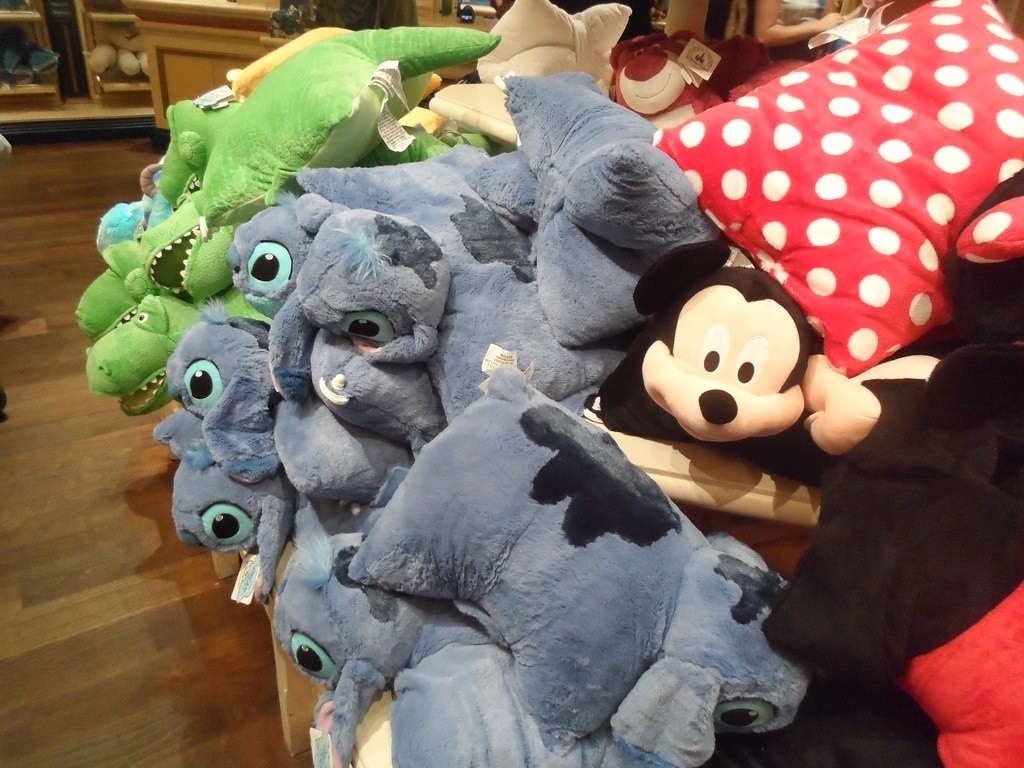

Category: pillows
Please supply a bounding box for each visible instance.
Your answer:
[299,0,1024,426]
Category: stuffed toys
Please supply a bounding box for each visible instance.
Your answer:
[89,23,150,76]
[0,27,58,86]
[0,0,30,11]
[75,0,1024,768]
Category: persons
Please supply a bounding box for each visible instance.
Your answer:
[747,0,876,63]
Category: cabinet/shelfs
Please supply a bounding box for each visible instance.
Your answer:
[1,1,61,105]
[73,1,150,103]
